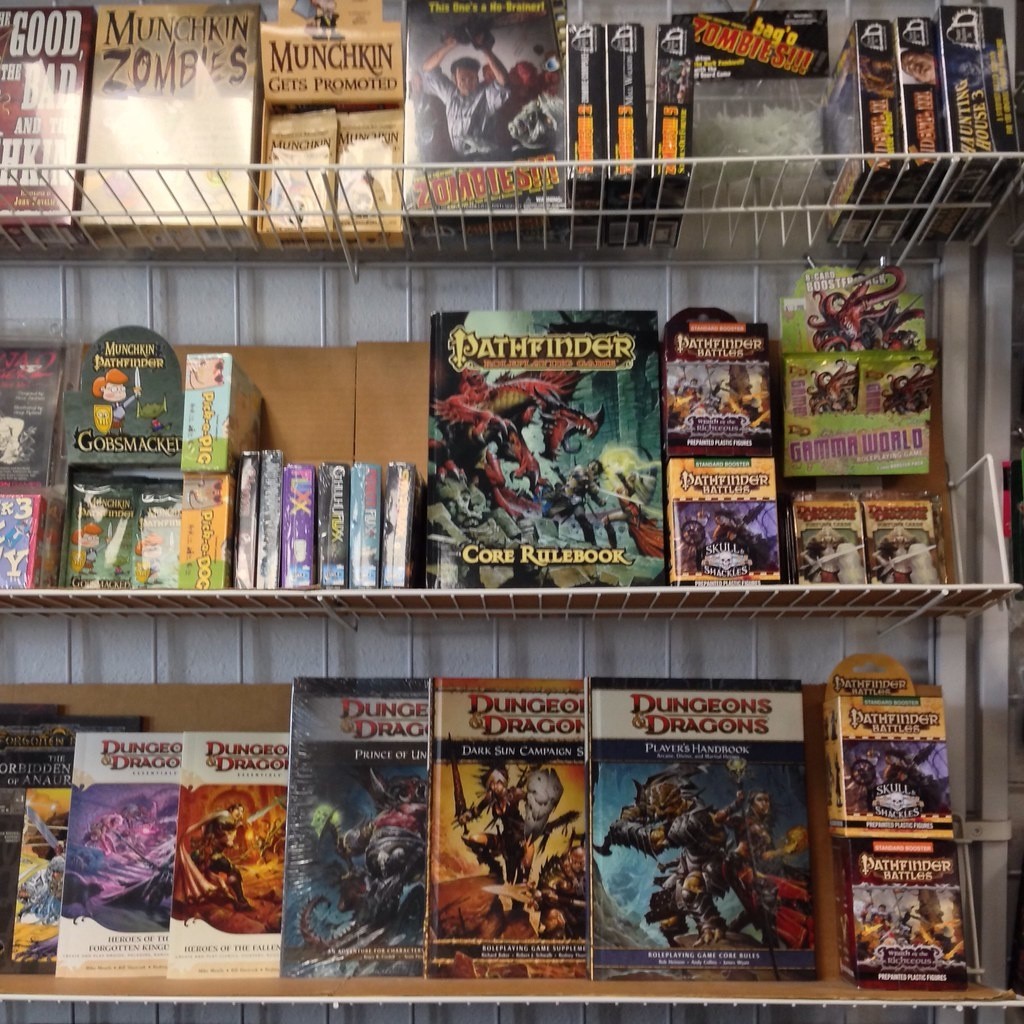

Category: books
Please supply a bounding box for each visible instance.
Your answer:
[1,5,265,249]
[0,677,814,984]
[422,310,665,586]
[403,0,566,238]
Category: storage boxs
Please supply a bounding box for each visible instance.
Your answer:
[659,307,773,463]
[785,490,957,588]
[57,326,183,588]
[257,0,408,249]
[822,652,955,840]
[0,347,74,589]
[424,310,666,586]
[233,451,260,589]
[179,473,237,589]
[603,23,646,246]
[0,6,98,245]
[567,23,606,248]
[904,6,1024,241]
[780,268,931,477]
[1,676,819,980]
[819,19,903,243]
[80,4,267,248]
[866,17,951,242]
[380,462,426,589]
[402,0,570,244]
[317,463,351,589]
[643,25,694,248]
[667,459,780,584]
[283,465,318,588]
[257,450,284,588]
[350,463,382,588]
[831,837,967,991]
[181,353,261,472]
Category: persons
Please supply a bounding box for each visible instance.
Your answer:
[872,524,919,586]
[420,30,513,161]
[803,527,851,583]
[901,49,938,83]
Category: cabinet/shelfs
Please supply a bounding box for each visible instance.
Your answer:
[0,0,1024,1024]
